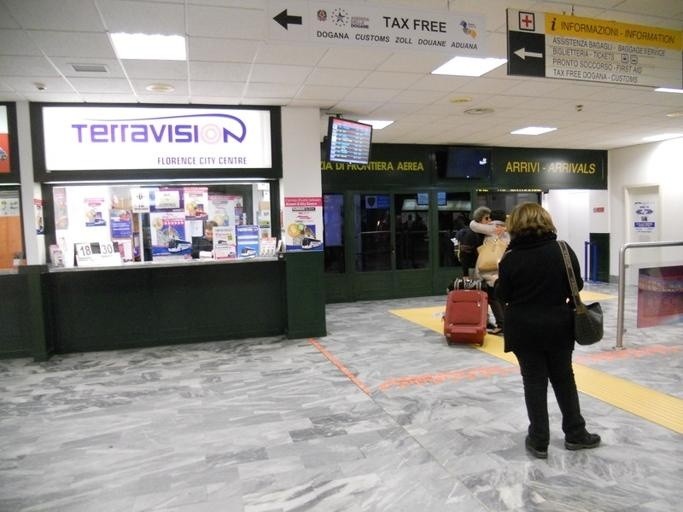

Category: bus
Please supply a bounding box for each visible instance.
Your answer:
[377,197,470,233]
[300,238,320,250]
[240,246,256,256]
[168,239,191,253]
[93,219,106,226]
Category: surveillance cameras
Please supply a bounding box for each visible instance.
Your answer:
[35,85,47,92]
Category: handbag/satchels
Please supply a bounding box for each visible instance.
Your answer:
[477,242,508,269]
[448,277,487,292]
[575,303,603,344]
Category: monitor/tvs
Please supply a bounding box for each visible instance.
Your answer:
[444,145,493,181]
[324,116,373,164]
[190,236,213,258]
[417,192,447,206]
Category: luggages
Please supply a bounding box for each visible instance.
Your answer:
[444,277,488,346]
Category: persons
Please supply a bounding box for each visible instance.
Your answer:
[446,206,507,291]
[371,210,467,267]
[469,209,512,334]
[191,219,217,259]
[493,200,601,458]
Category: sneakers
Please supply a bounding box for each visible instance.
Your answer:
[565,433,601,450]
[526,436,547,458]
